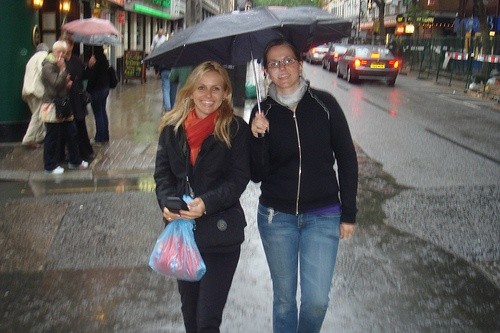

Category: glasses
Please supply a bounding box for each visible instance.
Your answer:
[266,56,298,68]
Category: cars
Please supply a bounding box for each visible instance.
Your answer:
[303,45,331,65]
[335,44,400,86]
[321,45,348,73]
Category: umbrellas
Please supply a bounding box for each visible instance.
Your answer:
[142,6,352,139]
[61,18,119,55]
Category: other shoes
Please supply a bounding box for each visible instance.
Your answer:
[21,141,41,149]
[68,161,89,169]
[44,166,65,175]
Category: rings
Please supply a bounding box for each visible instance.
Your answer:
[169,218,171,220]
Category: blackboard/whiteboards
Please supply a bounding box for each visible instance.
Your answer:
[123,50,145,80]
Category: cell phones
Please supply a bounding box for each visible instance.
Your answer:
[165,197,189,214]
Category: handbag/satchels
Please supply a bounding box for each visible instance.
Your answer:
[147,217,207,283]
[108,66,119,90]
[55,101,73,120]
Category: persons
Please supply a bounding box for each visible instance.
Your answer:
[41,41,89,174]
[20,42,50,146]
[150,28,167,52]
[154,61,249,333]
[58,36,95,159]
[250,39,358,333]
[83,46,111,141]
